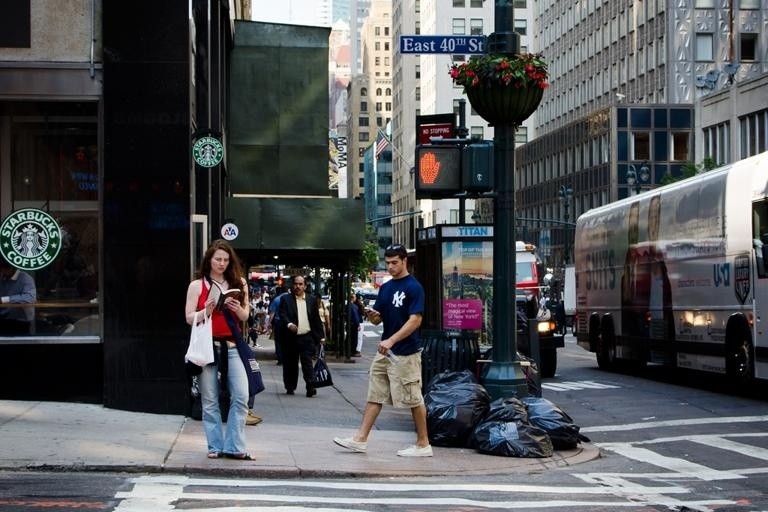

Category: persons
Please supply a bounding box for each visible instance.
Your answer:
[333,244,434,458]
[279,275,326,397]
[556,298,566,334]
[644,193,679,368]
[620,201,646,361]
[184,238,258,461]
[248,286,370,366]
[0,253,37,337]
[222,394,261,425]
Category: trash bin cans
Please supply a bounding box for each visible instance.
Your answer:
[420,329,481,398]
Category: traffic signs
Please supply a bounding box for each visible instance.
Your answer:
[408,142,468,200]
[396,32,486,55]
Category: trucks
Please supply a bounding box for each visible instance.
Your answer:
[558,264,578,337]
[574,147,768,395]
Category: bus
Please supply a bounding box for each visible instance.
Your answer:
[515,239,543,320]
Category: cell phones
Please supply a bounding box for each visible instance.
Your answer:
[364,306,380,316]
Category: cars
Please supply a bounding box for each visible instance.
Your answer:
[249,265,392,304]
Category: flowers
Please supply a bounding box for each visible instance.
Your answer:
[450,53,551,93]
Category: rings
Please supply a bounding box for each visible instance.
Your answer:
[234,303,236,306]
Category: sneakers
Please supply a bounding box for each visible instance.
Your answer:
[396,444,433,457]
[333,437,368,453]
[351,354,361,357]
[356,351,360,353]
[248,409,262,423]
[287,390,294,394]
[344,359,356,363]
[307,389,316,397]
[246,414,260,425]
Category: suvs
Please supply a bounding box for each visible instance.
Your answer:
[510,288,565,381]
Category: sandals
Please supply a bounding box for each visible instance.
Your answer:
[208,451,223,458]
[224,452,256,460]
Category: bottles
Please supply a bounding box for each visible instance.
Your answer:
[384,349,400,366]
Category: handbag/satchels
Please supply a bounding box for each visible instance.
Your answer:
[313,344,334,388]
[235,338,265,398]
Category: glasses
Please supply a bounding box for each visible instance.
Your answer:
[386,245,405,251]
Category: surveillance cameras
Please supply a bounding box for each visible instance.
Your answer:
[612,91,626,102]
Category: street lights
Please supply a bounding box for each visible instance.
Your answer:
[554,183,575,265]
[622,157,650,196]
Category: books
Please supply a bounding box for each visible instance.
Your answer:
[206,280,241,313]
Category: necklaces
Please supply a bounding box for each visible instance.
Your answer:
[210,276,227,285]
